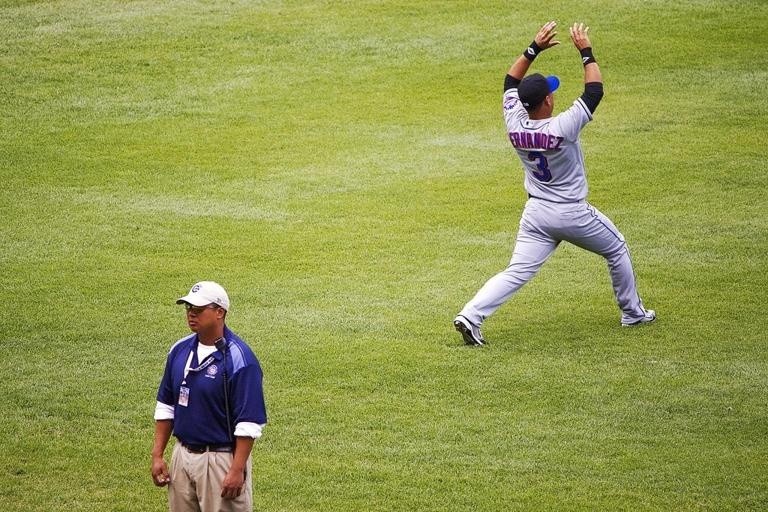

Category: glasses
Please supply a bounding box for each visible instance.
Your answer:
[184,303,208,314]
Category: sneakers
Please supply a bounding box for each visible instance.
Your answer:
[620,309,657,328]
[454,315,486,346]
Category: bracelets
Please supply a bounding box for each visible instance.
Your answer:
[521,41,543,61]
[579,46,597,67]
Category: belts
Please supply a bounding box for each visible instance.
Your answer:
[179,440,235,454]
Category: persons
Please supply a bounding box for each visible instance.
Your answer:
[152,280,268,512]
[452,19,657,344]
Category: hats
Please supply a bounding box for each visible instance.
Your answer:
[517,73,560,108]
[175,281,230,312]
